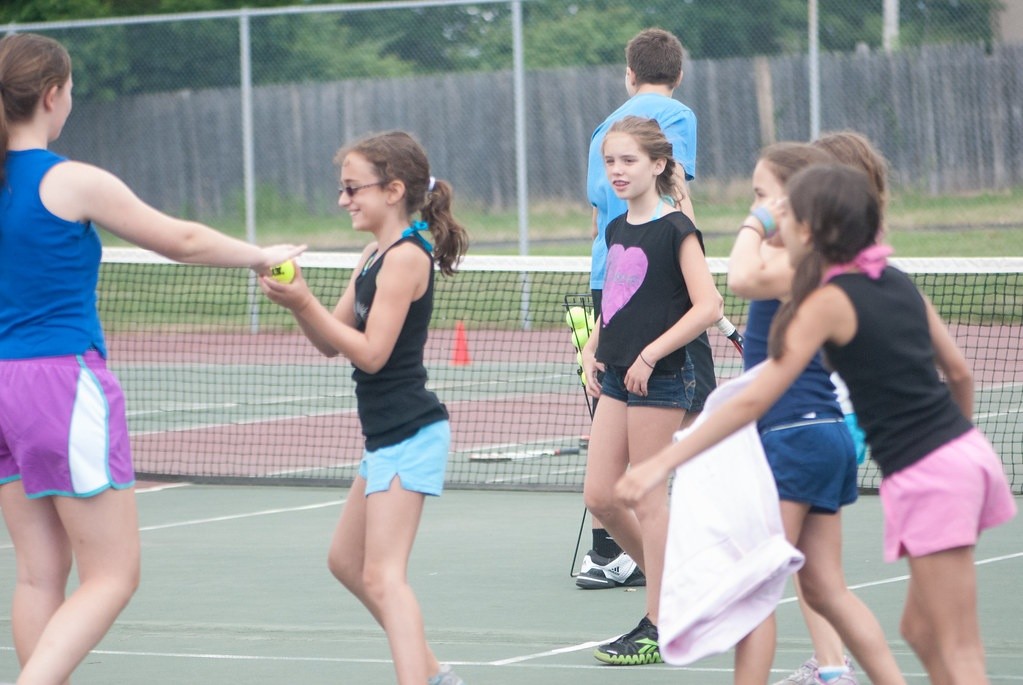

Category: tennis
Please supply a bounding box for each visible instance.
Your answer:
[266,259,295,283]
[566,306,595,385]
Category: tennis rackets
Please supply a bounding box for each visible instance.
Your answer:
[717,316,745,358]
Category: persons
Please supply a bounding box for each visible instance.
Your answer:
[0,31,308,685]
[582,114,724,664]
[259,130,471,685]
[577,30,718,588]
[613,163,1019,685]
[725,133,907,685]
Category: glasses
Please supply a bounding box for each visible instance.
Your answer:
[339,182,385,197]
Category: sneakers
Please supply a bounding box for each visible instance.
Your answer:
[593,617,664,665]
[576,548,646,589]
[772,655,859,685]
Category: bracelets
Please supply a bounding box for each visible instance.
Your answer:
[753,206,774,238]
[640,353,654,369]
[738,225,764,239]
